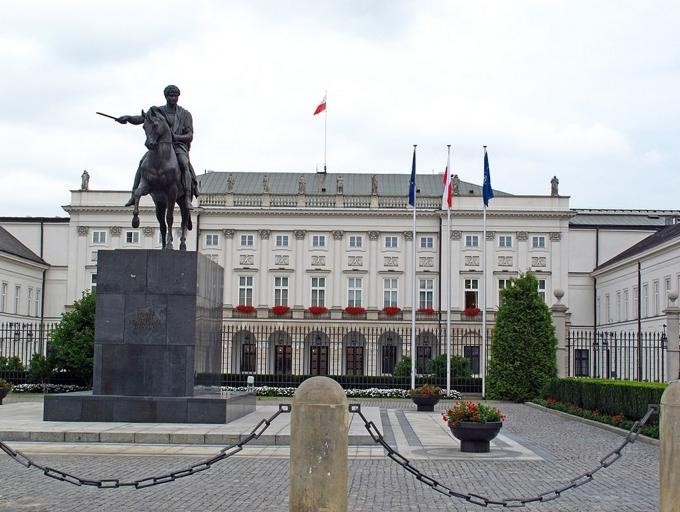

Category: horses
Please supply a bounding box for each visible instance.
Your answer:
[131,106,194,253]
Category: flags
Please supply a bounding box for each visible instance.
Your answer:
[408,148,415,208]
[312,94,326,115]
[441,157,453,209]
[483,151,494,207]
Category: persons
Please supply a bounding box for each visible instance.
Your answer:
[550,176,560,197]
[371,174,378,195]
[225,173,235,194]
[263,174,270,193]
[336,175,344,194]
[114,85,195,211]
[81,169,90,191]
[296,174,307,194]
[452,174,460,193]
